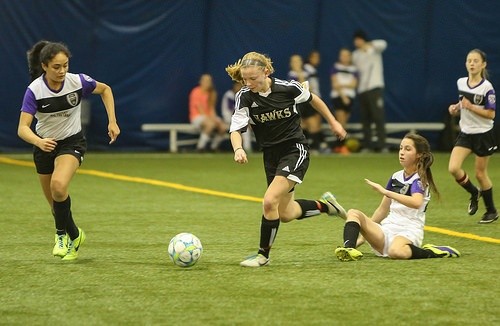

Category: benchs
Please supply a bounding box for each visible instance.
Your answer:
[140,124,445,156]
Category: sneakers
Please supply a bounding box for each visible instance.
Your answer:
[61,227,86,261]
[239,252,271,268]
[317,190,349,222]
[467,186,482,216]
[53,232,70,257]
[479,211,499,224]
[422,243,462,259]
[334,245,364,262]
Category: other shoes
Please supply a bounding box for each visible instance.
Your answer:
[333,145,349,156]
[379,145,389,154]
[195,148,207,154]
[210,148,222,154]
[360,145,375,154]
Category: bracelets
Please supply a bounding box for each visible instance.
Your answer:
[235,148,243,152]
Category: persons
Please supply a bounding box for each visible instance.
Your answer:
[335,134,460,261]
[331,49,358,154]
[449,48,499,224]
[18,40,120,260]
[190,75,226,154]
[222,79,252,152]
[352,32,389,153]
[224,52,348,266]
[289,52,330,154]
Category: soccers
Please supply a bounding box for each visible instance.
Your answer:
[168,233,203,268]
